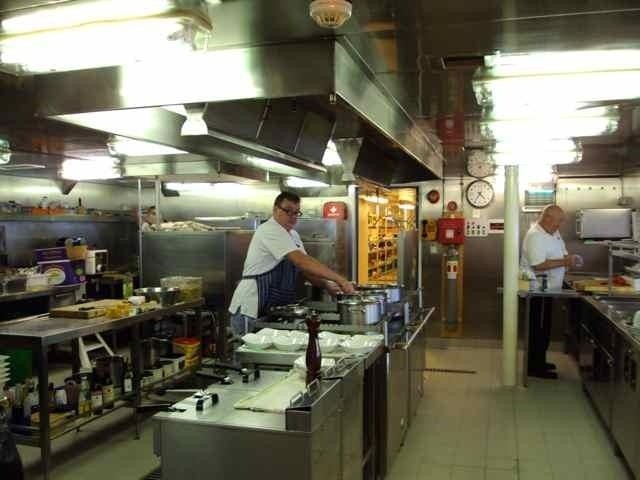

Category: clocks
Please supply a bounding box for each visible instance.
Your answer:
[466,148,494,178]
[465,180,495,208]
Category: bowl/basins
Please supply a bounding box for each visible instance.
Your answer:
[133,286,181,306]
[243,326,384,355]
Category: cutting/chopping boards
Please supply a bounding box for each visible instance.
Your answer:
[51,299,128,319]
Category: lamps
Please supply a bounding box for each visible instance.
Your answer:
[180,102,208,136]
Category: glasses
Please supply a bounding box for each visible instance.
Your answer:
[278,205,303,217]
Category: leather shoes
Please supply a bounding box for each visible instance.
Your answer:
[528,363,557,378]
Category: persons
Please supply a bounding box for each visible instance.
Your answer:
[228,192,353,365]
[142,206,156,232]
[522,205,577,379]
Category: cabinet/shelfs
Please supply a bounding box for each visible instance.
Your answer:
[357,187,416,283]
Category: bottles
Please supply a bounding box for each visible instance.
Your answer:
[65,234,88,257]
[4,364,133,426]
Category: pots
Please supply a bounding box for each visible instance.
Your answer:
[335,285,400,325]
